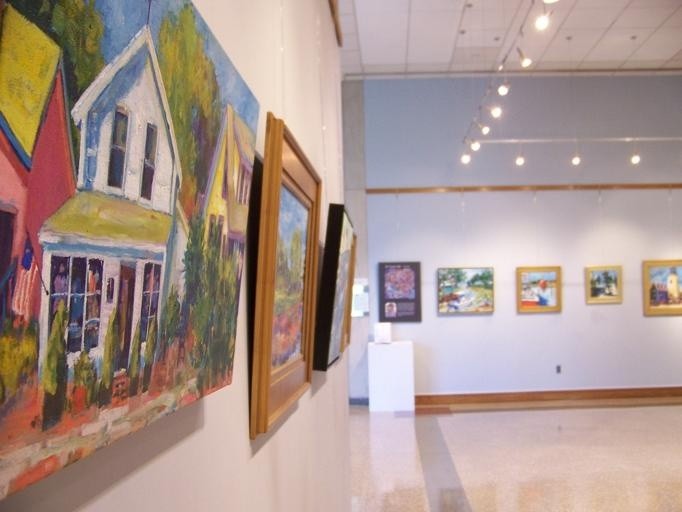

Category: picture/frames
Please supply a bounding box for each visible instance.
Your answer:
[249,108,359,442]
[641,258,682,317]
[516,266,562,313]
[436,266,495,315]
[585,264,623,304]
[377,261,421,322]
